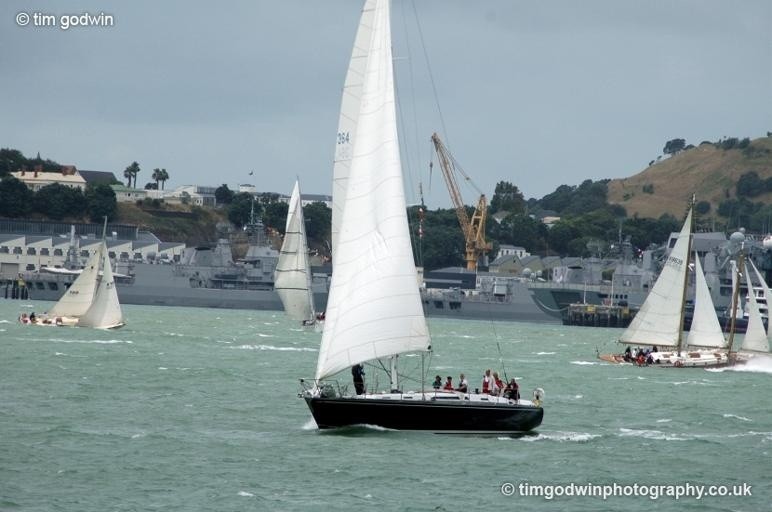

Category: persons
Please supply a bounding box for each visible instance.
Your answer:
[433,369,520,400]
[352,365,365,395]
[624,345,658,366]
[21,313,37,324]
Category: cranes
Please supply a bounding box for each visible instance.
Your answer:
[430,132,494,272]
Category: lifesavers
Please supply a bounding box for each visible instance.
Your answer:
[673,360,680,368]
[533,387,545,404]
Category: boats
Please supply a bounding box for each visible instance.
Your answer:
[18,203,328,324]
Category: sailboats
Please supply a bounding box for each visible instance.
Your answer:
[297,0,545,436]
[596,191,772,369]
[22,216,126,331]
[272,173,329,333]
